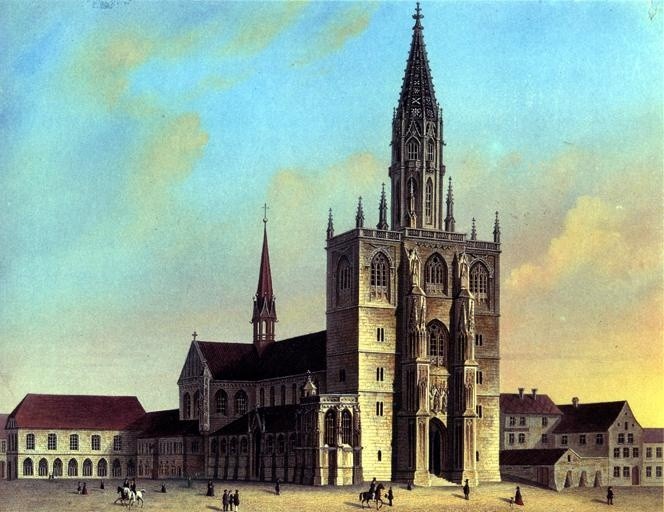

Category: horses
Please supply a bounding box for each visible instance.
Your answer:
[359,482,385,510]
[123,487,146,510]
[114,486,138,507]
